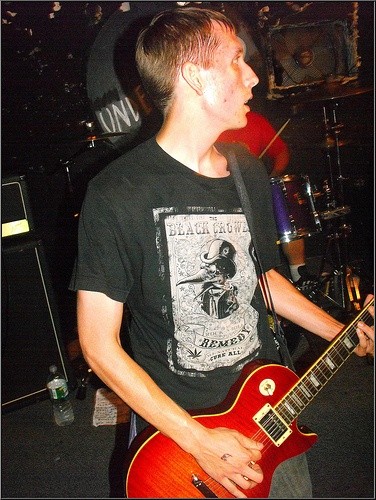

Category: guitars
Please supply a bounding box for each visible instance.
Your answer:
[116,297,376,498]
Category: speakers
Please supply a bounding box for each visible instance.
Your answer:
[0,240,74,415]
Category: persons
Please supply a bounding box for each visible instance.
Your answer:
[69,7,374,500]
[213,107,290,174]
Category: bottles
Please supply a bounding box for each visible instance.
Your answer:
[47,365,74,426]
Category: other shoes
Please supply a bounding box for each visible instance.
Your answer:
[293,264,319,287]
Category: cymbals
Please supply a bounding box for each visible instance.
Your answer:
[276,82,374,105]
[71,132,131,144]
[298,133,373,151]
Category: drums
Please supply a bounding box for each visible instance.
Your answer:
[269,173,324,243]
[313,186,353,220]
[266,308,309,366]
